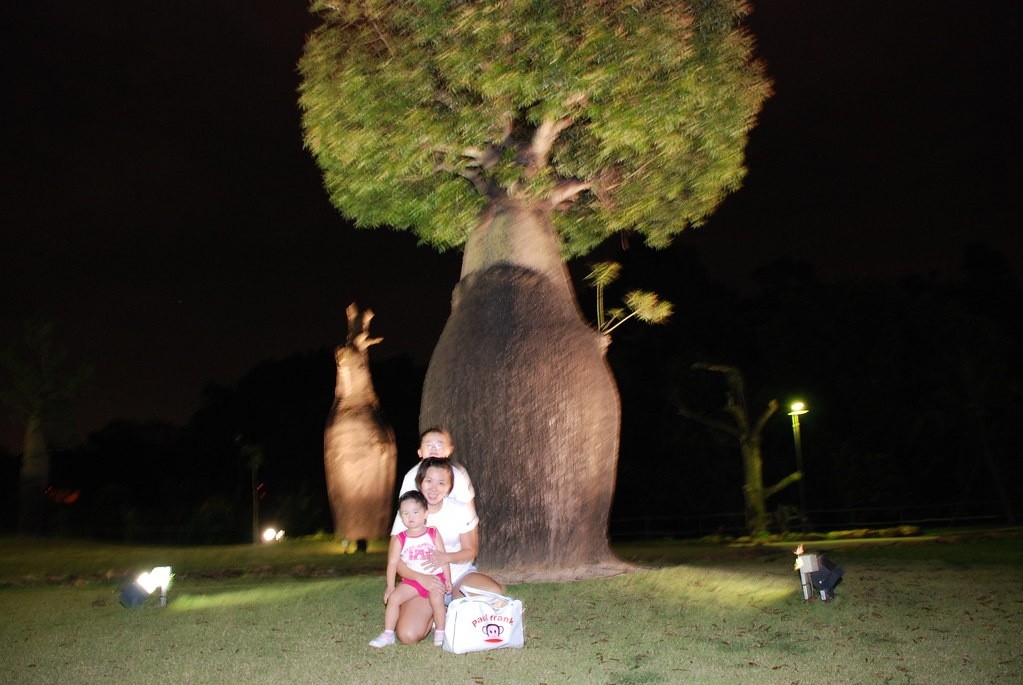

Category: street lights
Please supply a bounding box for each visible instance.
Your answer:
[787,402,810,534]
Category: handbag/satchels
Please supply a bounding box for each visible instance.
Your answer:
[441,585,524,655]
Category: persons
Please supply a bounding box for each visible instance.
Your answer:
[398,427,476,511]
[369,489,455,650]
[387,458,504,645]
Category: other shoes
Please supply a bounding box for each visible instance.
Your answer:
[434,629,444,646]
[368,632,395,648]
